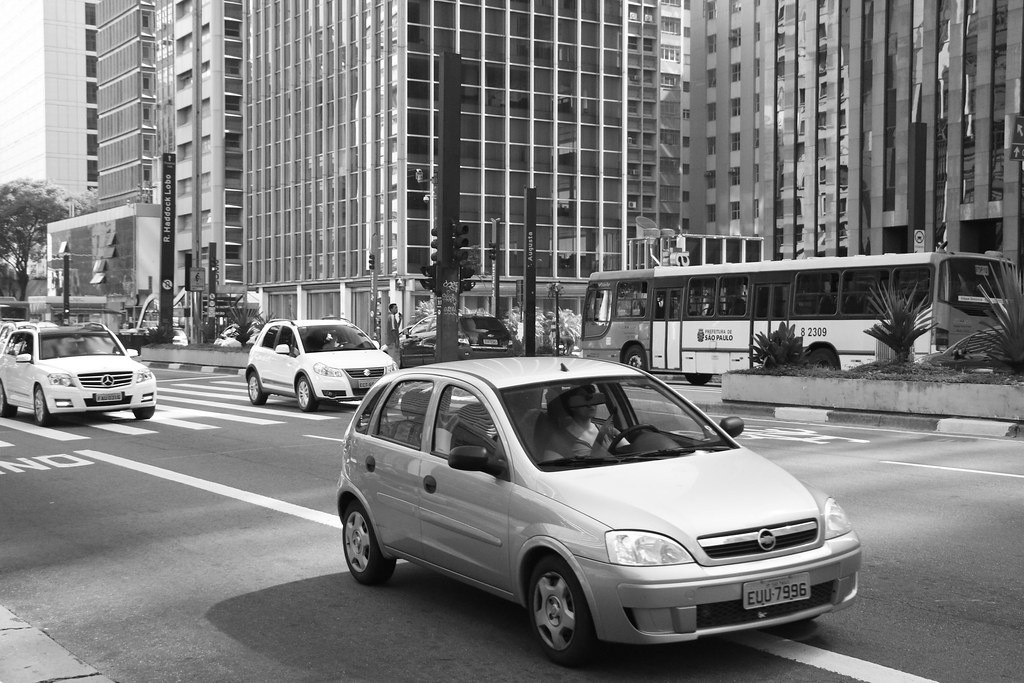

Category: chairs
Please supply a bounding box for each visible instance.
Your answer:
[533,390,574,461]
[636,284,924,316]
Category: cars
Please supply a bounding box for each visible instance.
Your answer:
[338,354,863,664]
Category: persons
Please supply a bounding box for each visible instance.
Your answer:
[544,385,631,462]
[385,303,402,369]
[559,337,574,354]
[323,333,343,350]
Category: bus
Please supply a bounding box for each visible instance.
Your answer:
[576,248,1016,382]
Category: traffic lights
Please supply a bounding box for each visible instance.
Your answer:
[453,223,469,261]
[461,268,476,293]
[488,242,496,260]
[420,264,436,290]
[431,227,438,261]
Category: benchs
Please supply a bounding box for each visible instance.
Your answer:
[402,389,531,451]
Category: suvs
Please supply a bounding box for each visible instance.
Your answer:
[1,321,158,427]
[380,309,514,372]
[242,316,399,412]
